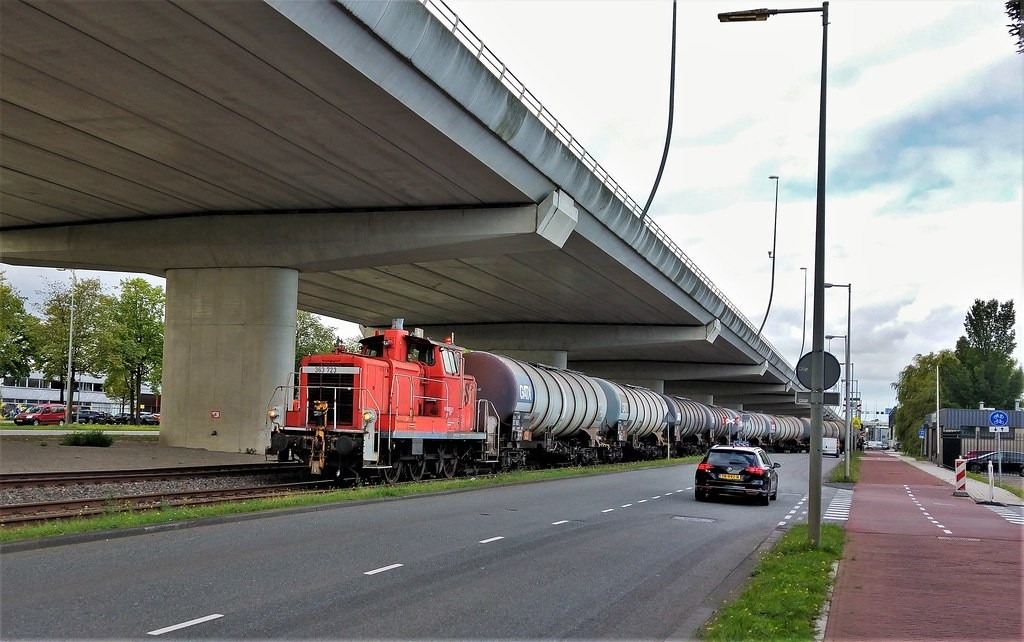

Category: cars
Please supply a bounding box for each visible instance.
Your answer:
[966,450,1024,478]
[961,450,999,459]
[694,444,781,506]
[71,410,161,427]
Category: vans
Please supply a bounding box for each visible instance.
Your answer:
[823,437,843,458]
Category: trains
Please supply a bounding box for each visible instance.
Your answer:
[264,318,850,486]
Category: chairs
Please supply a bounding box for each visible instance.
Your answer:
[720,452,732,459]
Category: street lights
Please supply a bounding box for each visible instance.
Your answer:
[935,364,952,466]
[55,268,75,426]
[754,174,781,337]
[841,378,859,452]
[838,362,854,452]
[849,391,861,438]
[824,282,853,480]
[717,2,832,549]
[794,268,807,373]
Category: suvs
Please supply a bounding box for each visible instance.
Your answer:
[13,403,66,427]
